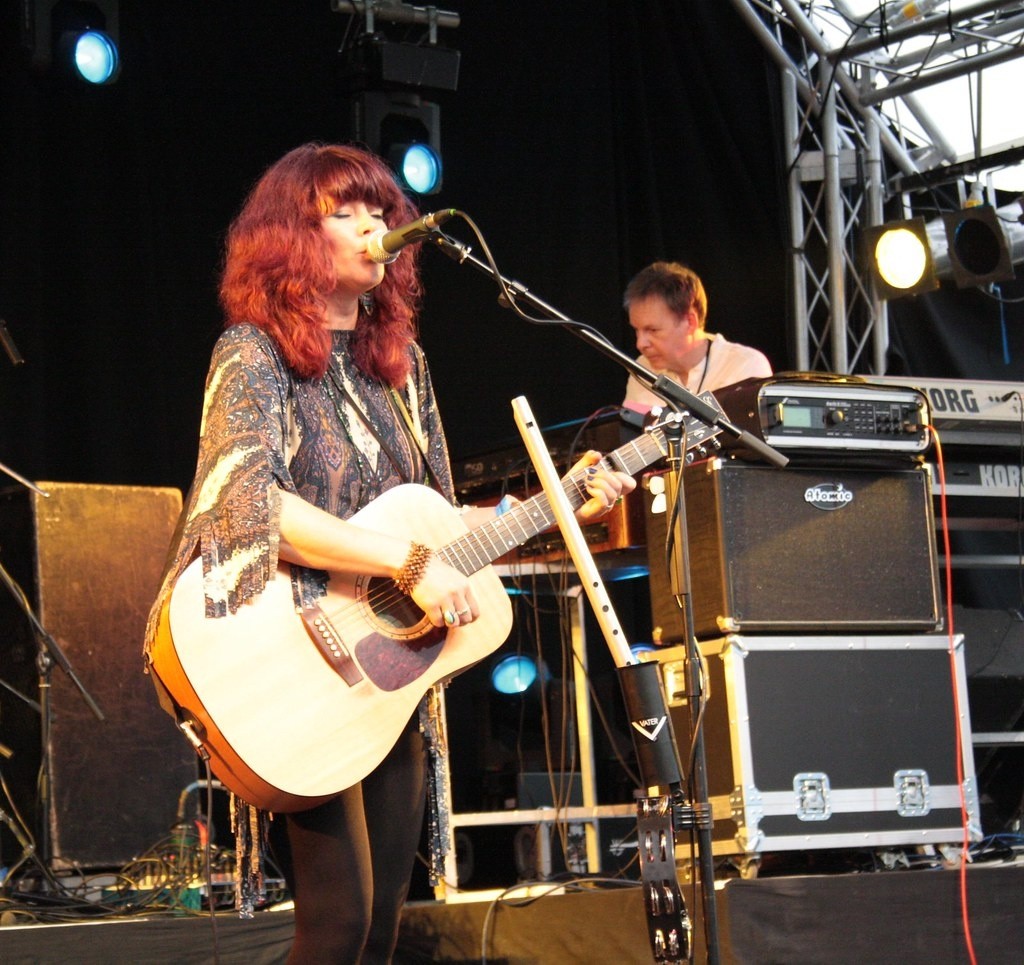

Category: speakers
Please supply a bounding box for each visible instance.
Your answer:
[642,456,943,649]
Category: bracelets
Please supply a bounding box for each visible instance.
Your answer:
[392,541,432,597]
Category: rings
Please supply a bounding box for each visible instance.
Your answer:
[444,610,455,623]
[459,608,472,614]
[617,495,623,505]
[608,505,614,511]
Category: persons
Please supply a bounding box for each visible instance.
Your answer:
[615,263,773,412]
[143,144,637,965]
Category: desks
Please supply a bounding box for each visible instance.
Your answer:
[417,554,644,902]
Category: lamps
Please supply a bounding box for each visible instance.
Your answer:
[942,203,1024,293]
[865,217,939,301]
[387,141,442,196]
[487,647,537,692]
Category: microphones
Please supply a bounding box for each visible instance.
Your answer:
[365,208,455,264]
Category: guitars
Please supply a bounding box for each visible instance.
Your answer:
[142,387,735,810]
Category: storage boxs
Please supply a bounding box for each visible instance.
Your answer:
[650,635,985,889]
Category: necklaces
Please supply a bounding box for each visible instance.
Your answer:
[321,365,364,511]
[696,337,713,393]
